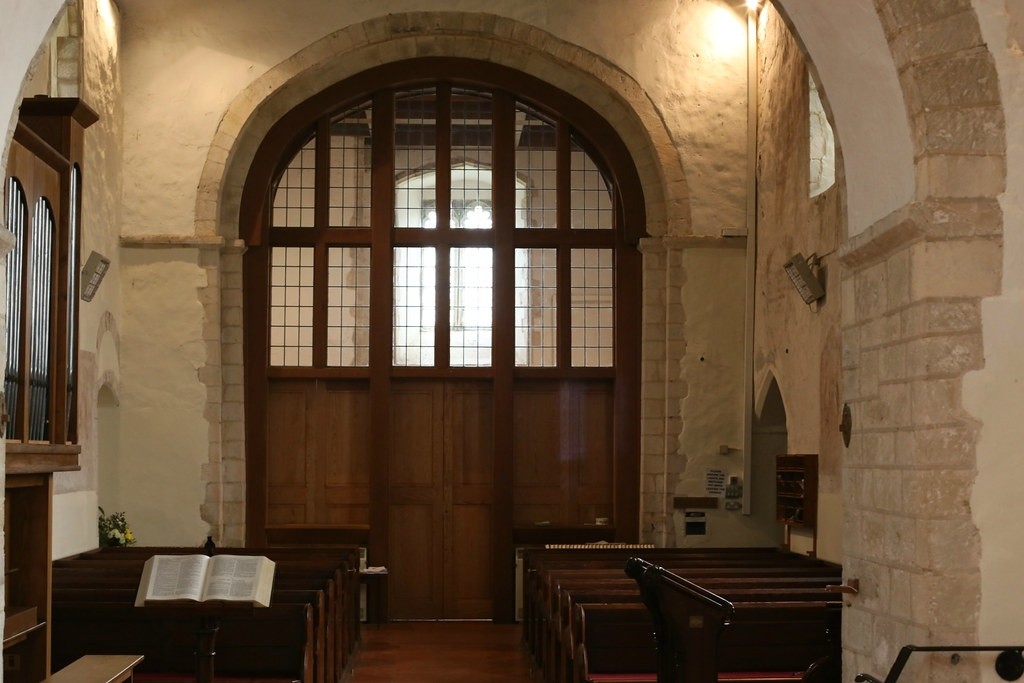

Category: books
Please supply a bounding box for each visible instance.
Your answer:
[134,555,276,609]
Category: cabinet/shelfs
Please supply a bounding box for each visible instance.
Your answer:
[775,453,817,528]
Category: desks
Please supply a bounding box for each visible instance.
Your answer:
[361,575,384,630]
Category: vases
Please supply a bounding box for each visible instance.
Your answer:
[107,538,119,546]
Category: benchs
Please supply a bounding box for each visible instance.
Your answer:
[523,546,842,683]
[52,546,360,683]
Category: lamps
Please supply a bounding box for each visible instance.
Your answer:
[783,252,825,305]
[81,250,111,302]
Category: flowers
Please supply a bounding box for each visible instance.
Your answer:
[98,506,137,545]
